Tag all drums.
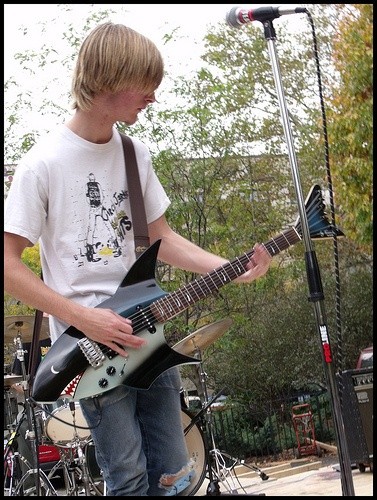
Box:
[44,401,92,449]
[83,406,210,495]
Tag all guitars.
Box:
[31,185,345,404]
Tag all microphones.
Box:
[226,7,307,29]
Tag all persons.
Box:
[4,22,272,497]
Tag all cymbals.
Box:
[171,317,233,354]
[4,315,51,343]
[4,376,27,386]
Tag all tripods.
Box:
[189,336,269,495]
[4,325,104,496]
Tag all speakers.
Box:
[335,366,375,466]
